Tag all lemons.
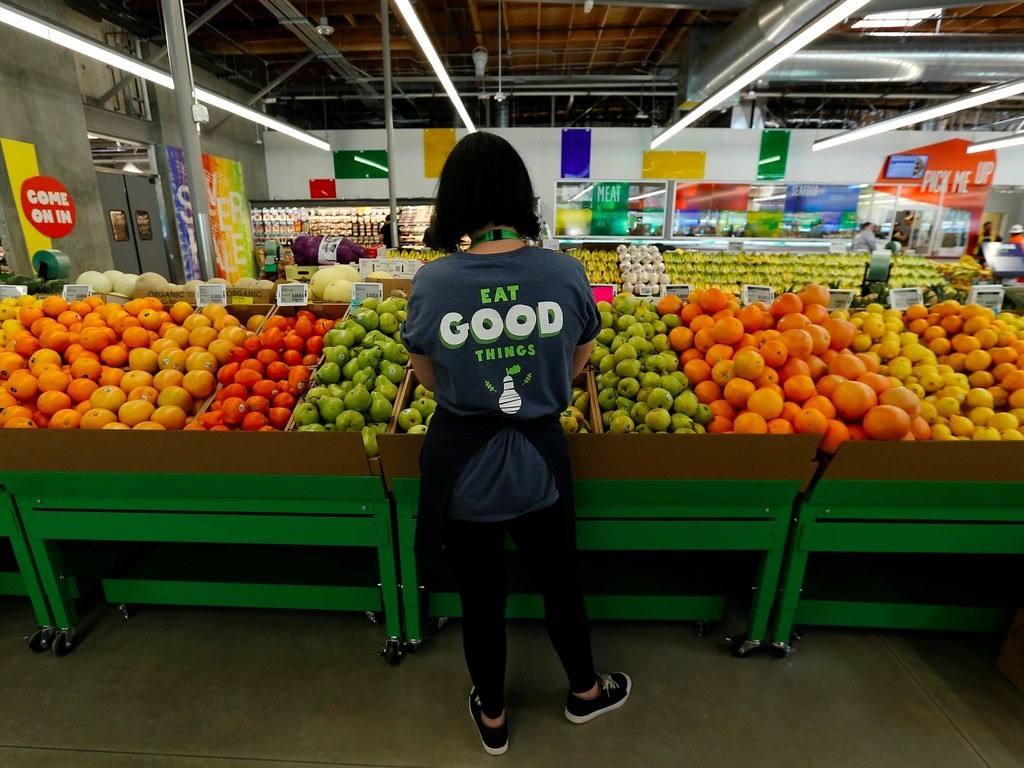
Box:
[829,310,1024,441]
[866,303,884,314]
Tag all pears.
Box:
[559,293,713,434]
[293,297,436,458]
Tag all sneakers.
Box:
[468,683,507,755]
[565,671,632,724]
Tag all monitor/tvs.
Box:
[885,155,929,179]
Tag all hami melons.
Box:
[76,265,392,303]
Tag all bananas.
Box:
[387,249,445,263]
[557,249,956,297]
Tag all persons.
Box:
[851,223,880,254]
[380,213,402,249]
[1003,224,1023,287]
[399,133,631,754]
[973,220,1003,265]
[686,227,697,236]
[808,218,828,238]
[891,212,913,246]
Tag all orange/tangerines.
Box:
[657,285,928,451]
[0,295,339,431]
[903,300,1024,435]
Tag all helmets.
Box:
[1008,223,1024,233]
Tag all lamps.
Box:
[0,0,1024,172]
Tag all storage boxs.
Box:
[163,303,414,433]
[570,359,605,434]
